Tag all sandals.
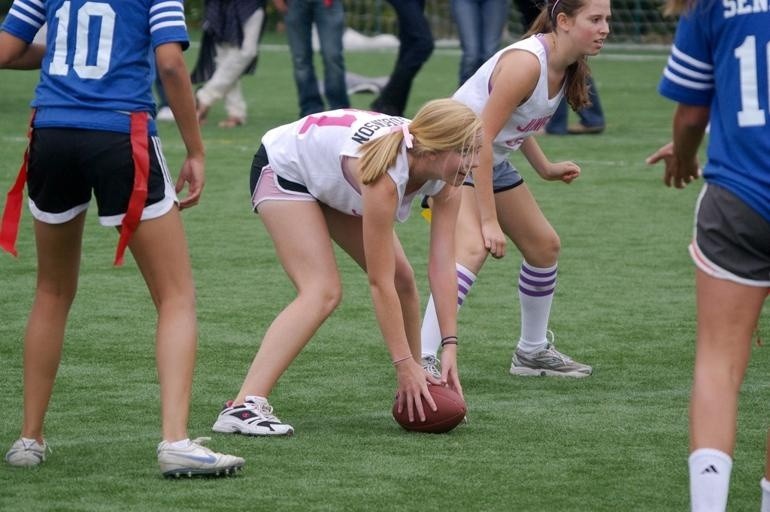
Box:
[217,118,248,129]
[193,87,209,124]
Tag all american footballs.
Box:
[393,384,467,433]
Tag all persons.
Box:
[209,98,485,437]
[642,0,770,511]
[416,0,610,383]
[0,1,244,480]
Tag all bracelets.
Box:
[442,340,459,348]
[441,335,458,343]
[391,354,413,367]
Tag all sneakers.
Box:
[420,354,442,384]
[156,440,244,477]
[212,395,293,437]
[565,122,604,135]
[508,342,592,379]
[5,436,48,467]
